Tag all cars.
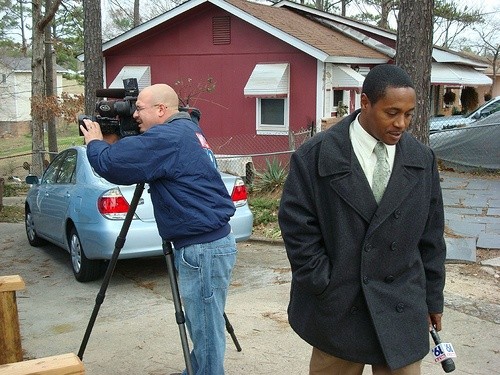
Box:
[428,95,500,133]
[24,147,253,283]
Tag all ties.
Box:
[372,142,390,206]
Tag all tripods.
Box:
[76,183,243,375]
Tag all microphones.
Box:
[429,328,457,373]
[96,89,134,99]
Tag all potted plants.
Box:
[441,91,456,116]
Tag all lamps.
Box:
[353,65,360,73]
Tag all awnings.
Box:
[108,66,151,92]
[244,63,289,97]
[431,62,493,87]
[332,64,365,94]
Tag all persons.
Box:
[278,63,446,375]
[81,83,236,375]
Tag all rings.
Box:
[88,127,92,130]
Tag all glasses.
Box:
[136,104,167,112]
